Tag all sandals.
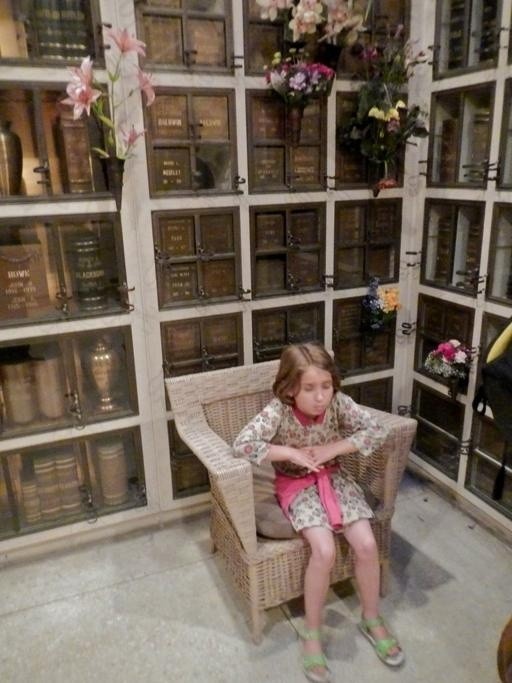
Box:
[355,613,407,667]
[295,616,334,683]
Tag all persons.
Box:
[229,339,405,683]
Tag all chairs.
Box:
[166,352,421,647]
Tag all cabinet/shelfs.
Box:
[0,1,406,534]
[410,2,512,545]
